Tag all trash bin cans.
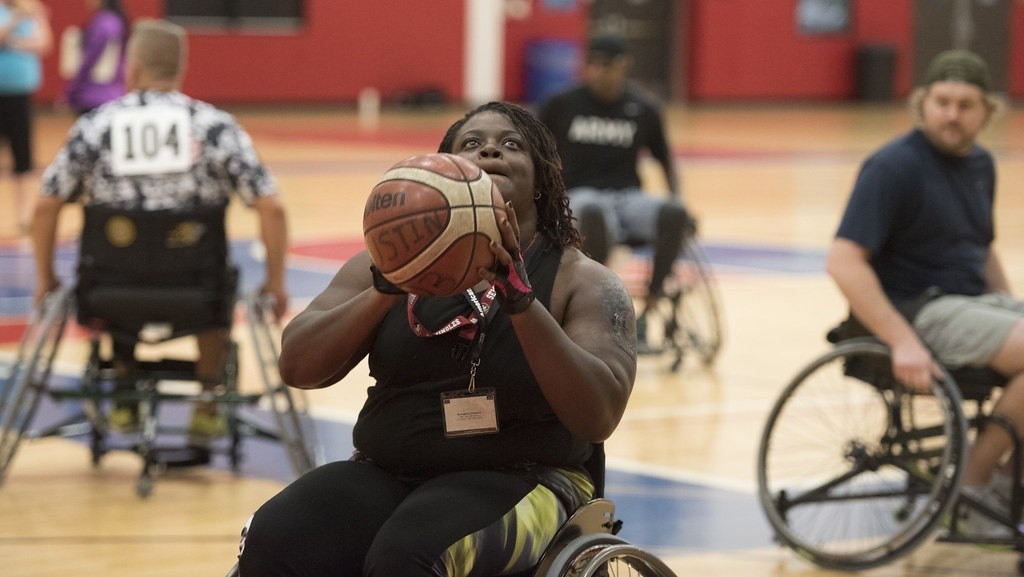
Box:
[854,42,896,103]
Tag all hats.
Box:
[924,49,997,88]
[585,36,624,57]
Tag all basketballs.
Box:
[360,153,510,297]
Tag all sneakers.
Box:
[104,407,138,430]
[188,415,226,433]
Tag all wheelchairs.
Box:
[756,310,1024,572]
[0,189,317,481]
[530,441,675,577]
[635,218,724,376]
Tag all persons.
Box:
[58,0,129,120]
[31,18,291,450]
[0,1,54,238]
[534,34,696,355]
[232,102,637,577]
[826,47,1024,528]
